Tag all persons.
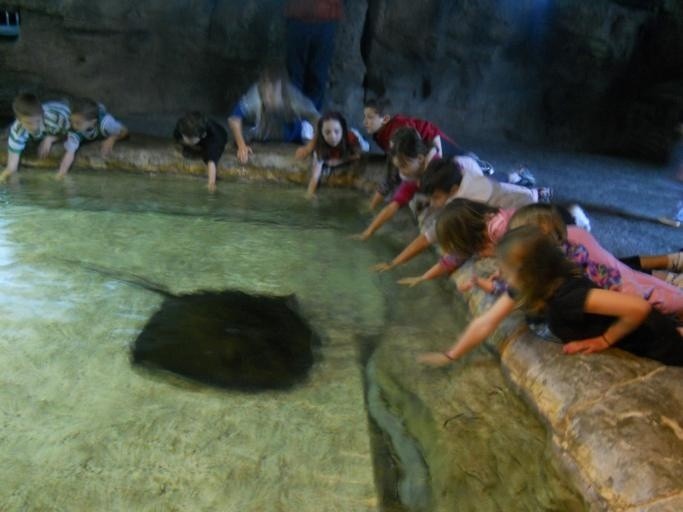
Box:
[457,205,682,334]
[305,108,369,203]
[231,73,323,168]
[345,137,556,243]
[359,125,555,213]
[369,159,593,273]
[395,198,683,319]
[616,249,682,288]
[0,92,72,186]
[172,110,229,194]
[362,96,495,180]
[415,223,683,374]
[50,96,128,175]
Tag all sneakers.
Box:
[476,156,494,174]
[569,202,591,231]
[539,186,554,205]
[515,166,535,187]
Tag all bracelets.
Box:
[386,263,395,267]
[442,351,454,361]
[602,333,611,348]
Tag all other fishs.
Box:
[66,258,318,395]
